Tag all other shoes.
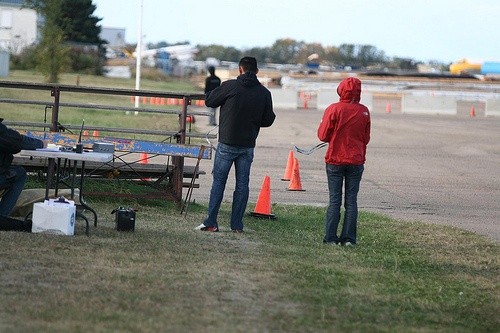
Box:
[344,242,352,246]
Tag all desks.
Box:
[11,151,112,235]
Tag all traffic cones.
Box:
[143,96,184,106]
[139,152,150,180]
[196,99,205,107]
[386,103,392,112]
[280,149,306,191]
[82,128,99,138]
[470,106,476,117]
[131,96,135,105]
[249,176,276,220]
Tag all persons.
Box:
[194,57,276,233]
[0,118,36,230]
[318,77,371,243]
[206,66,221,126]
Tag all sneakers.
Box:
[194,223,219,232]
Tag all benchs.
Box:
[5,154,209,190]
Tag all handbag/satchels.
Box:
[111,207,136,232]
[32,196,76,237]
